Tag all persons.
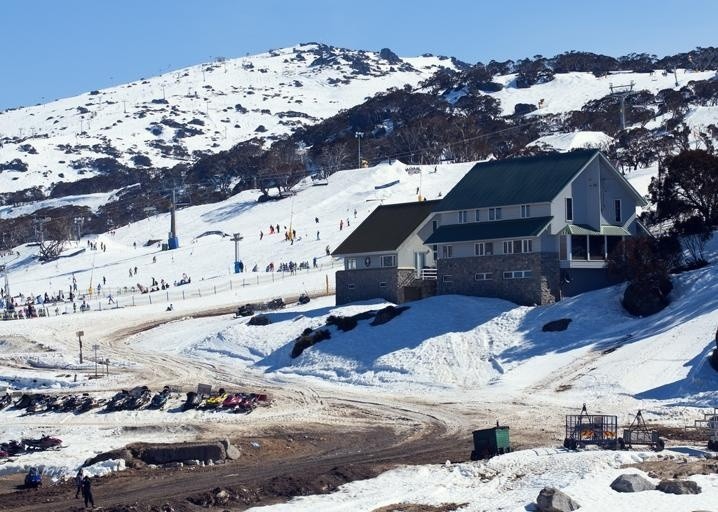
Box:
[133,242,136,249]
[17,251,20,256]
[69,273,78,293]
[82,475,95,508]
[326,246,330,256]
[315,217,319,223]
[340,220,343,230]
[86,228,116,253]
[0,288,114,321]
[439,192,442,196]
[157,241,160,248]
[354,209,357,217]
[167,304,174,311]
[129,267,192,294]
[75,467,87,500]
[317,231,320,239]
[252,257,317,275]
[416,187,427,201]
[259,224,296,245]
[435,167,437,172]
[153,255,157,263]
[102,276,106,284]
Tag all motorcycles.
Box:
[181,387,262,415]
[1,394,97,417]
[24,467,43,490]
[102,386,173,413]
[0,434,69,462]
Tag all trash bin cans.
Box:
[162,244,167,251]
[168,237,179,249]
[471,426,510,457]
